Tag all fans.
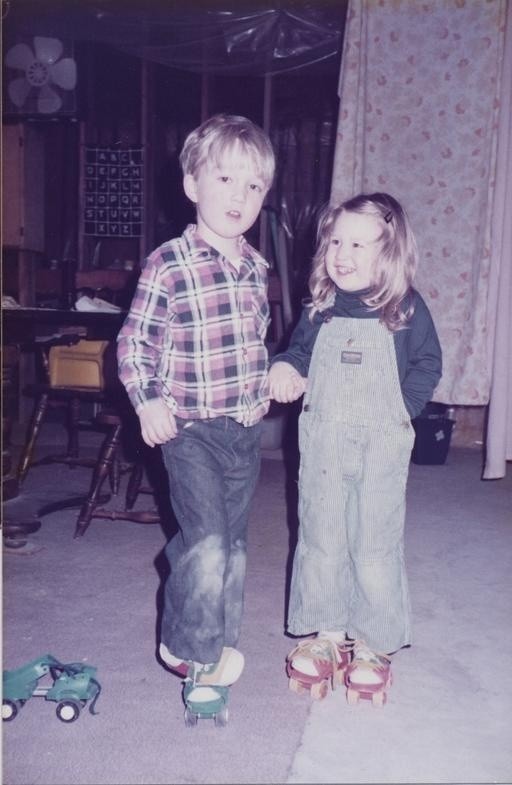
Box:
[4,36,81,115]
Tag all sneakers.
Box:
[180,675,232,728]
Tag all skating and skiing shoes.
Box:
[2,654,103,724]
[286,635,352,702]
[342,651,396,709]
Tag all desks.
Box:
[3,305,128,504]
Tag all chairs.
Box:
[16,268,179,535]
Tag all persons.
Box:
[267,192,443,686]
[117,111,305,690]
